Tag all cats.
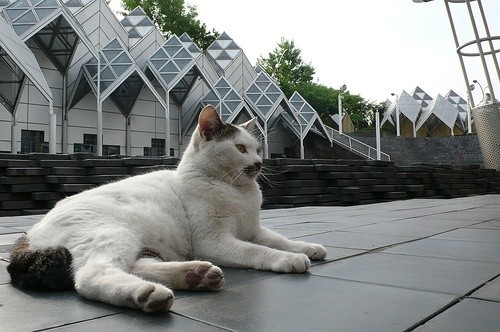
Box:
[7,105,328,314]
